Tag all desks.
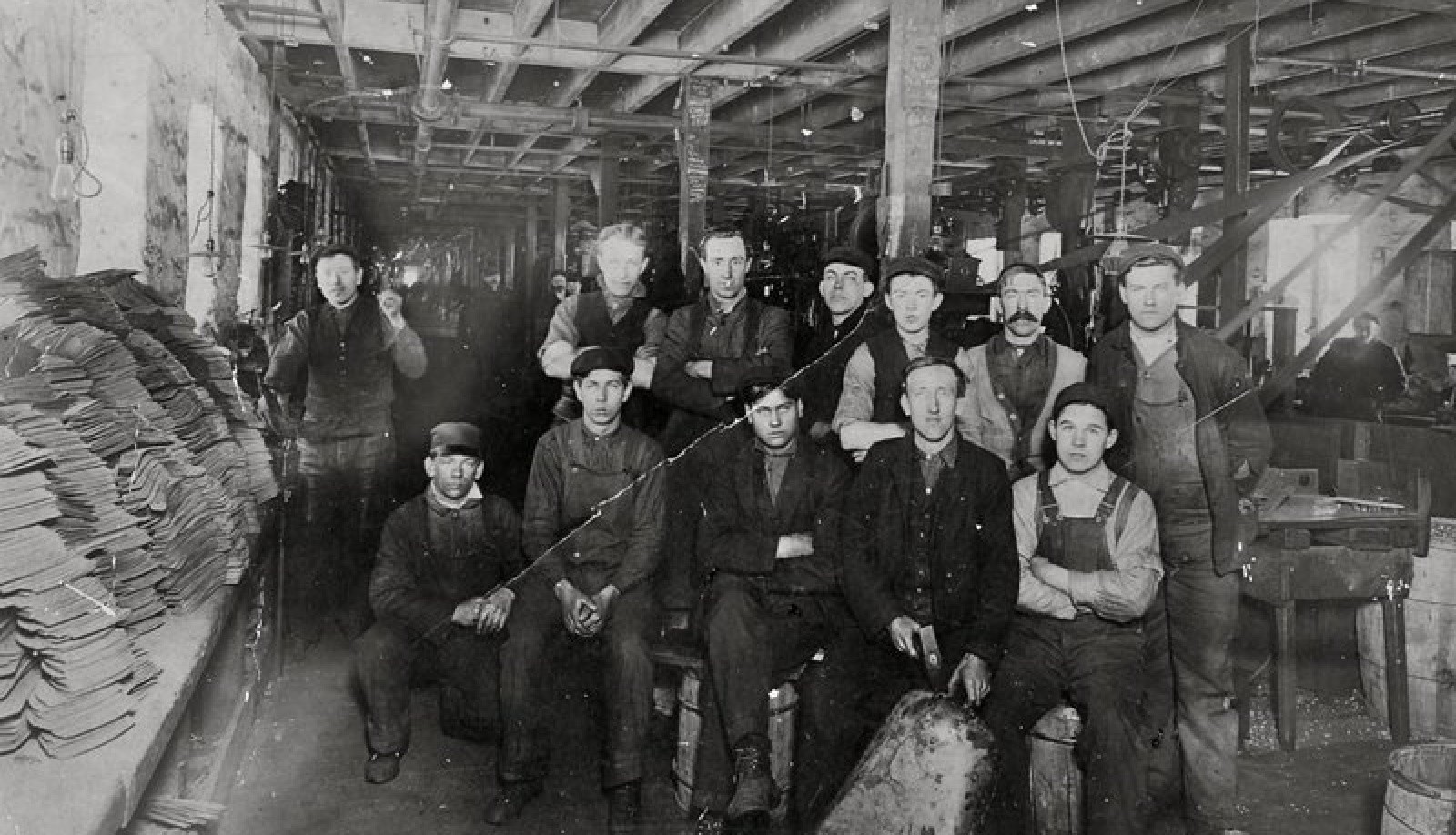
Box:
[1242,494,1426,752]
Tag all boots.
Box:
[727,739,772,826]
[696,812,730,835]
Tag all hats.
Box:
[571,347,635,378]
[887,256,947,287]
[734,364,808,408]
[428,422,483,458]
[1053,381,1120,431]
[821,247,875,276]
[1117,243,1185,275]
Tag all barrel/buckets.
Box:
[1026,704,1085,835]
[671,671,800,828]
[1353,517,1456,744]
[1382,741,1456,835]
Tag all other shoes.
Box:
[609,779,642,835]
[343,613,365,641]
[363,752,407,784]
[303,615,325,644]
[484,777,544,824]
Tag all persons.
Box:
[263,246,426,657]
[351,223,1273,834]
[1310,314,1408,421]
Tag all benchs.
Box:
[648,645,1085,835]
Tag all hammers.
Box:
[916,625,943,691]
